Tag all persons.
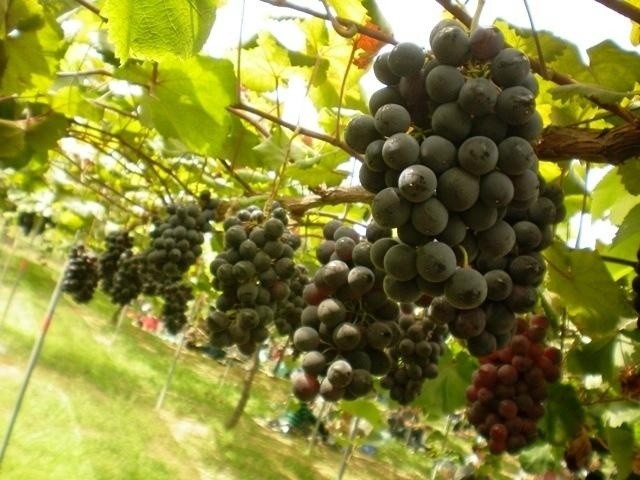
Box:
[395,405,432,454]
[455,443,489,479]
[287,402,343,455]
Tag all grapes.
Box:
[58,20,563,455]
